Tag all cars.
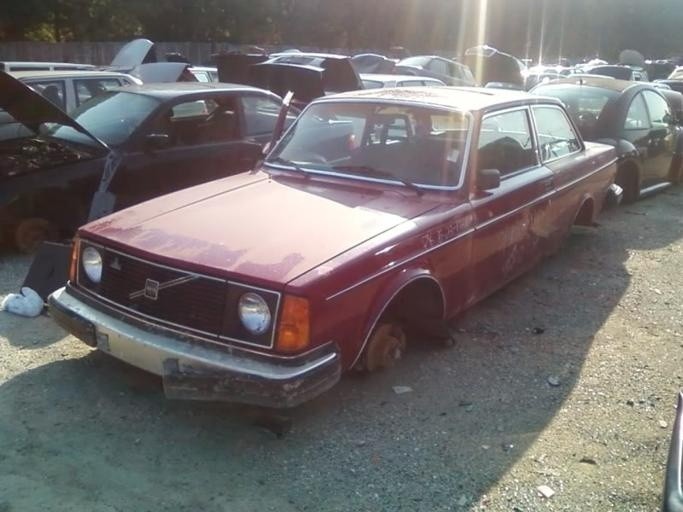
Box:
[528,77,683,203]
[47,86,621,410]
[0,69,363,255]
[1,36,683,122]
